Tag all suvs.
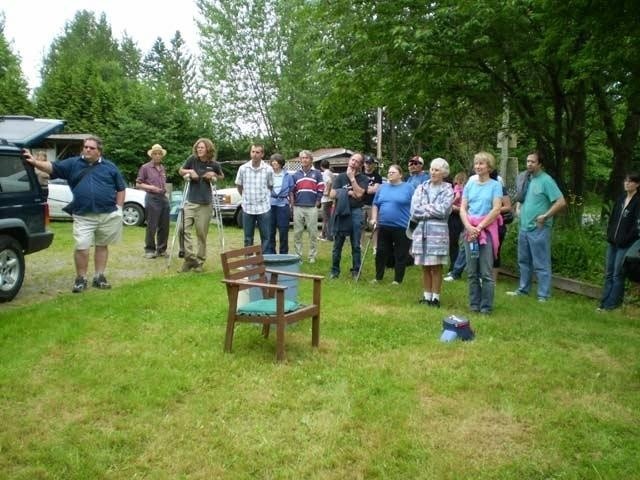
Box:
[0,118,65,304]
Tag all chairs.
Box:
[220,244,325,361]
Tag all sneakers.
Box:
[309,257,315,264]
[443,271,457,281]
[72,273,112,292]
[144,252,173,259]
[177,259,202,273]
[329,271,400,285]
[419,297,440,308]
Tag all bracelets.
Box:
[543,215,547,219]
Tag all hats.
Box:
[147,143,167,158]
[408,155,425,165]
[364,156,373,163]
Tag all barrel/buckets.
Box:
[247,255,301,303]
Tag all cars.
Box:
[213,170,347,230]
[0,168,147,228]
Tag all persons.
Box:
[175,137,224,274]
[329,152,455,309]
[22,137,126,293]
[34,151,50,203]
[444,152,512,313]
[593,171,640,316]
[505,151,568,306]
[135,144,170,259]
[234,144,335,264]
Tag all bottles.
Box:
[469,239,479,258]
